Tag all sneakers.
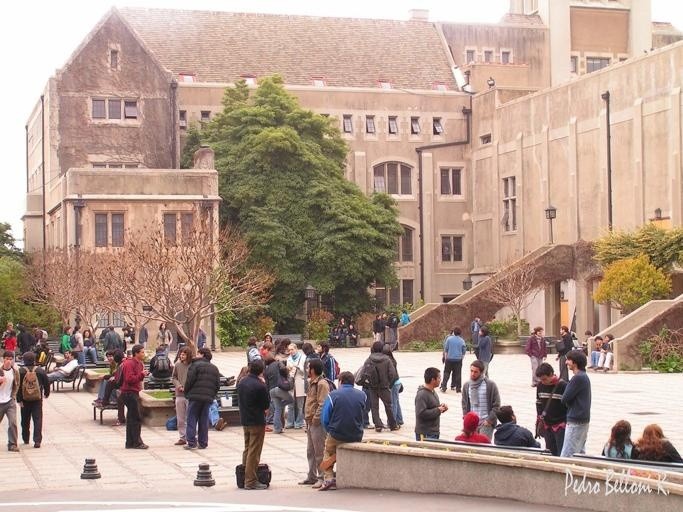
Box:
[265,420,308,434]
[93,398,110,408]
[244,483,268,490]
[319,453,336,471]
[298,479,336,491]
[125,444,148,450]
[215,417,227,431]
[174,439,207,451]
[7,446,20,452]
[23,436,29,445]
[34,442,40,449]
[111,420,125,427]
[439,386,462,393]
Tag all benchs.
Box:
[17,342,238,426]
[517,335,558,354]
[468,336,499,354]
[272,334,304,349]
[422,436,683,474]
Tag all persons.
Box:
[0,311,683,492]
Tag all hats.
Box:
[264,332,272,339]
[464,411,480,433]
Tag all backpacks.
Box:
[555,338,566,352]
[323,354,340,380]
[68,333,78,349]
[391,316,398,327]
[21,365,41,402]
[36,329,48,342]
[153,355,169,374]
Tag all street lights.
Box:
[544,205,556,242]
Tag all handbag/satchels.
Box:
[353,366,364,387]
[277,376,295,391]
[235,464,271,489]
[536,417,547,438]
[474,349,494,363]
[58,343,62,354]
[165,415,178,431]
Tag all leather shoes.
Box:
[364,423,404,432]
[531,383,538,387]
[587,366,611,372]
